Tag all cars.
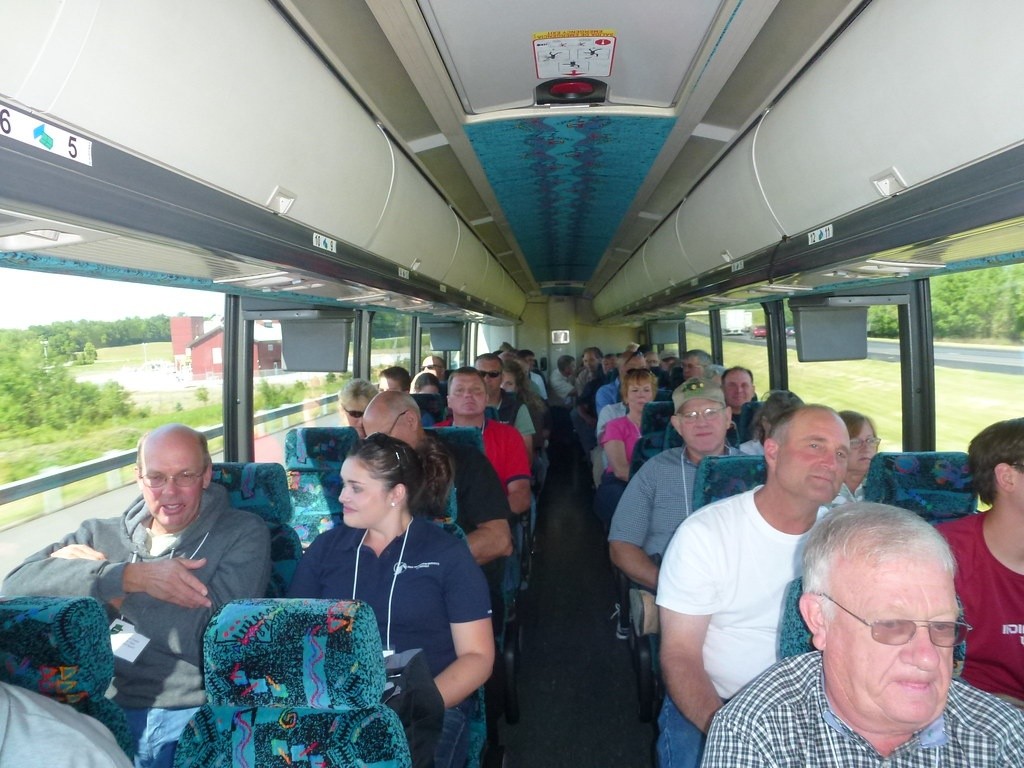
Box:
[786,327,795,337]
[753,325,767,339]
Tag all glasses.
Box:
[478,370,502,379]
[850,436,881,450]
[343,407,364,418]
[819,592,973,648]
[364,432,404,484]
[625,350,643,365]
[420,365,443,372]
[678,406,726,423]
[627,368,654,376]
[138,466,207,488]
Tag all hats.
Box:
[671,374,725,416]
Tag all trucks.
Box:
[741,312,752,332]
[720,309,745,336]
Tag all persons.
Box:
[0,680,134,768]
[361,390,514,635]
[570,338,760,532]
[409,341,548,448]
[283,430,498,768]
[928,415,1023,714]
[697,500,1024,768]
[0,425,275,768]
[338,378,379,440]
[829,409,883,507]
[378,366,411,394]
[548,355,578,451]
[606,376,754,595]
[430,365,532,515]
[739,389,804,456]
[653,402,851,768]
[475,352,536,461]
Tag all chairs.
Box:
[620,390,982,722]
[410,392,447,423]
[283,426,359,550]
[169,597,413,768]
[211,463,304,598]
[425,424,527,724]
[429,482,506,768]
[0,594,136,768]
